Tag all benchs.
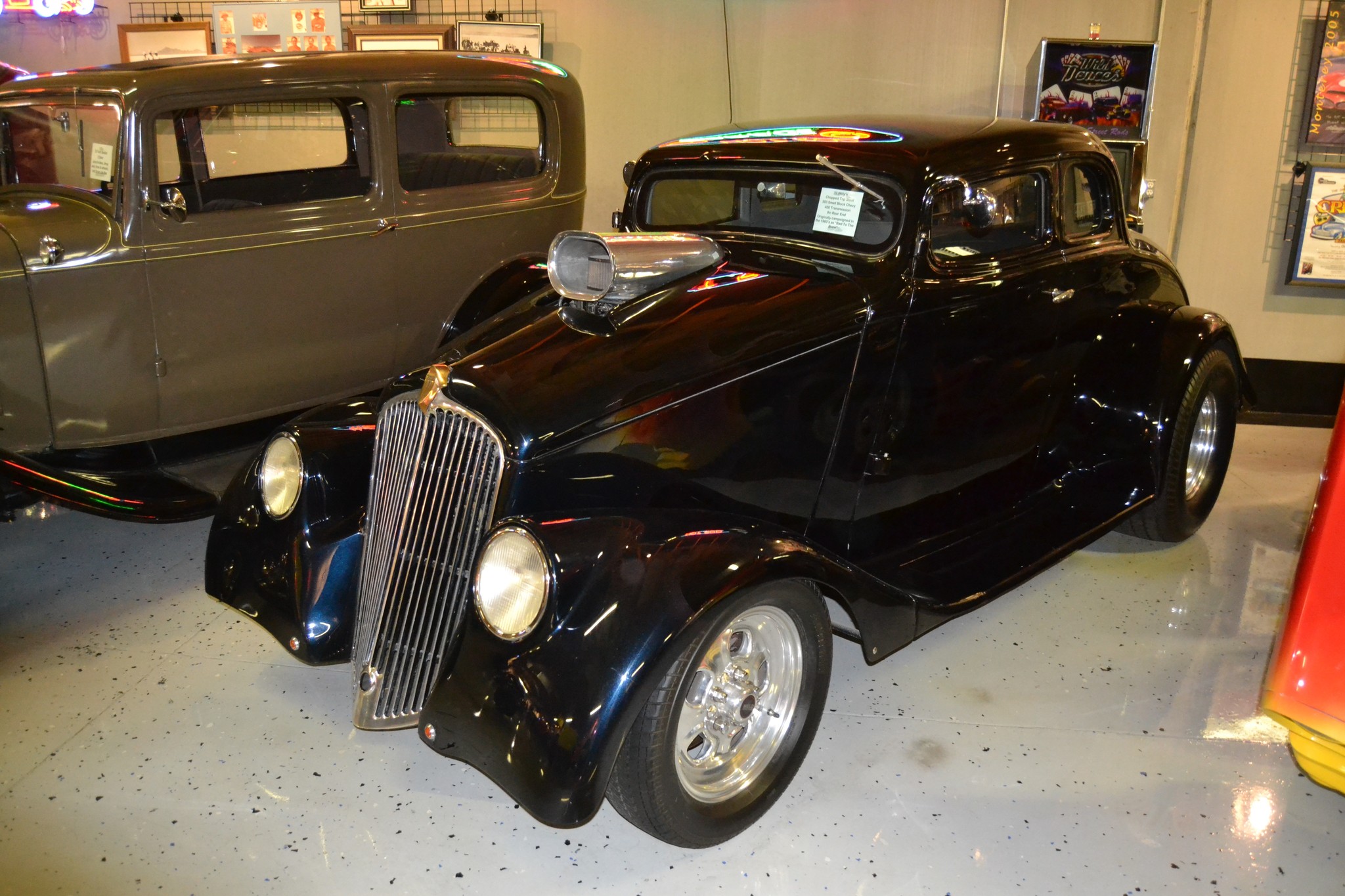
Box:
[399,152,531,190]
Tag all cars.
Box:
[0,45,588,512]
[202,113,1264,856]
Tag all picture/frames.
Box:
[1284,161,1345,287]
[1033,37,1159,141]
